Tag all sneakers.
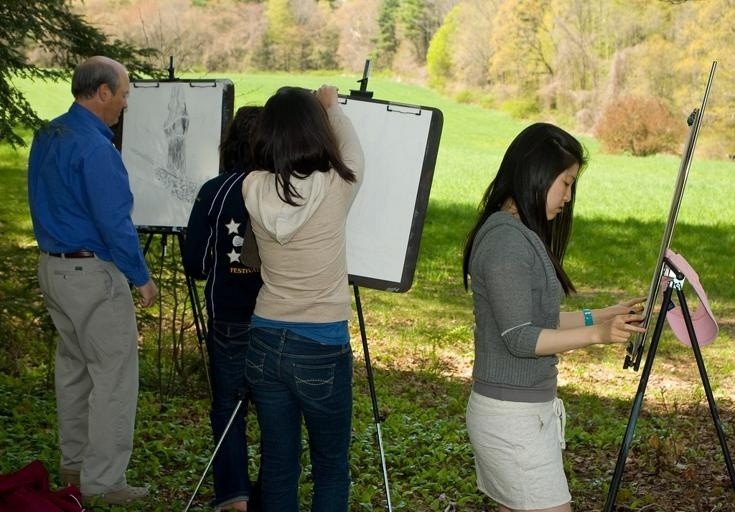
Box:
[58,465,149,507]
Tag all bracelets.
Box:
[582,308,594,327]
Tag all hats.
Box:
[660,248,721,350]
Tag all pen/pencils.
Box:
[625,311,635,325]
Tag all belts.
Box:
[41,249,95,259]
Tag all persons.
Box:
[180,105,272,511]
[240,81,369,511]
[459,122,649,511]
[26,54,160,505]
